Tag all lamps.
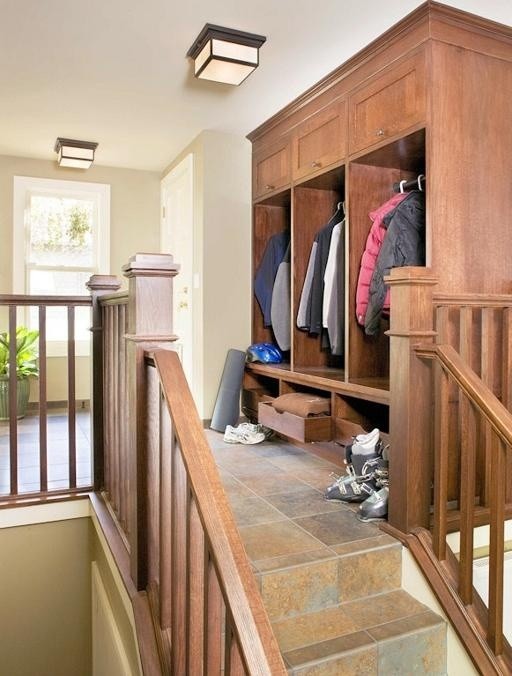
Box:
[185,21,267,88]
[51,136,98,171]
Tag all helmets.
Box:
[246,342,283,364]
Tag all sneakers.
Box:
[222,424,265,445]
[238,421,262,432]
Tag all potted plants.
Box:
[0,325,39,421]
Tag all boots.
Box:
[357,443,390,520]
[326,441,382,502]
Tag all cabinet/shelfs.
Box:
[240,0,512,503]
